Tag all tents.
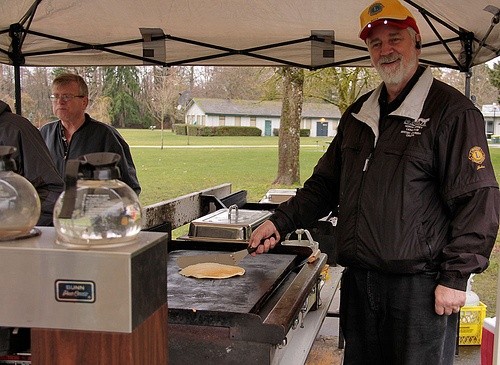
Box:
[0,1,500,116]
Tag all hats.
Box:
[357,0,416,38]
[359,16,420,40]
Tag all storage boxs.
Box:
[480,317,497,365]
[459,302,488,346]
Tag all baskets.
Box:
[458,300,487,346]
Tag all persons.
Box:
[0,99,65,354]
[39,74,141,196]
[248,0,500,365]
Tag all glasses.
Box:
[48,94,85,101]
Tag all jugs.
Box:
[52,153,143,248]
[0,145,41,240]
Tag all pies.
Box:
[179,262,245,279]
